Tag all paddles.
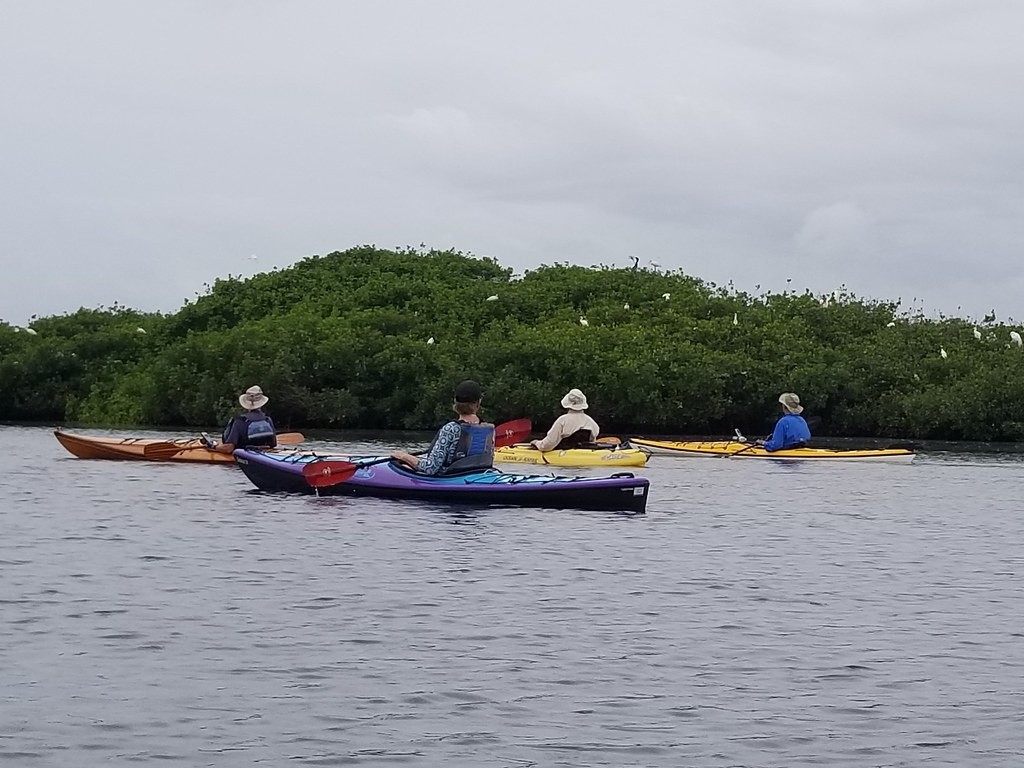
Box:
[597,435,621,446]
[142,433,302,462]
[304,418,533,486]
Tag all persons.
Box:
[207,385,277,453]
[391,379,495,475]
[755,392,811,452]
[530,389,599,451]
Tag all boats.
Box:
[492,444,654,467]
[627,435,918,465]
[53,426,349,464]
[233,447,651,513]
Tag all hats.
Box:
[455,380,487,403]
[239,385,268,409]
[778,392,804,414]
[561,389,588,410]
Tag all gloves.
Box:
[755,438,766,446]
[207,440,219,450]
